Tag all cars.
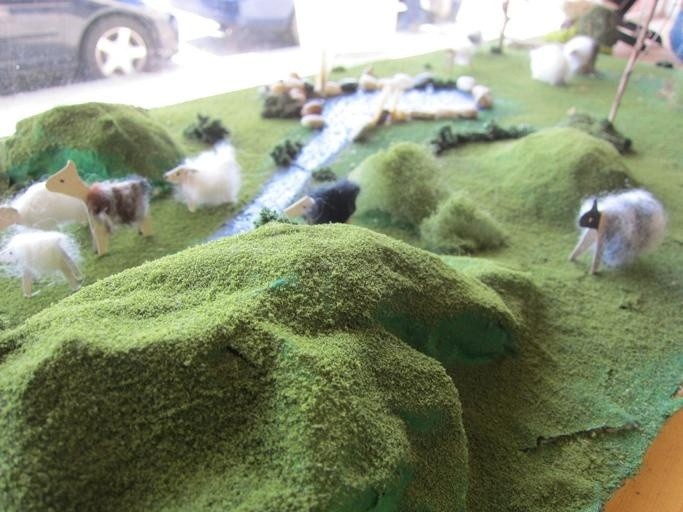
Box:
[1,0,179,98]
